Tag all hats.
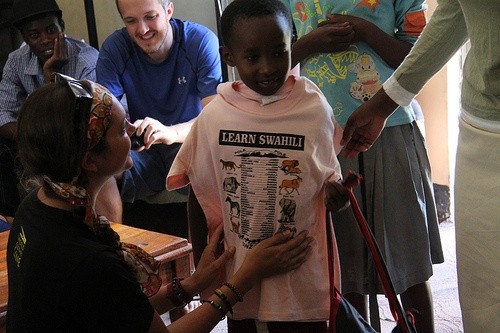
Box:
[15,0,62,26]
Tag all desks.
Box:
[0,221,197,333]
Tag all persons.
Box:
[280,0,444,333]
[340,0,500,333]
[96,0,223,228]
[4,73,314,333]
[165,0,359,333]
[0,0,130,143]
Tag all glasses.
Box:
[49,71,94,163]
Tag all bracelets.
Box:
[223,281,243,302]
[214,288,234,319]
[171,125,179,144]
[206,296,226,320]
[170,277,193,309]
